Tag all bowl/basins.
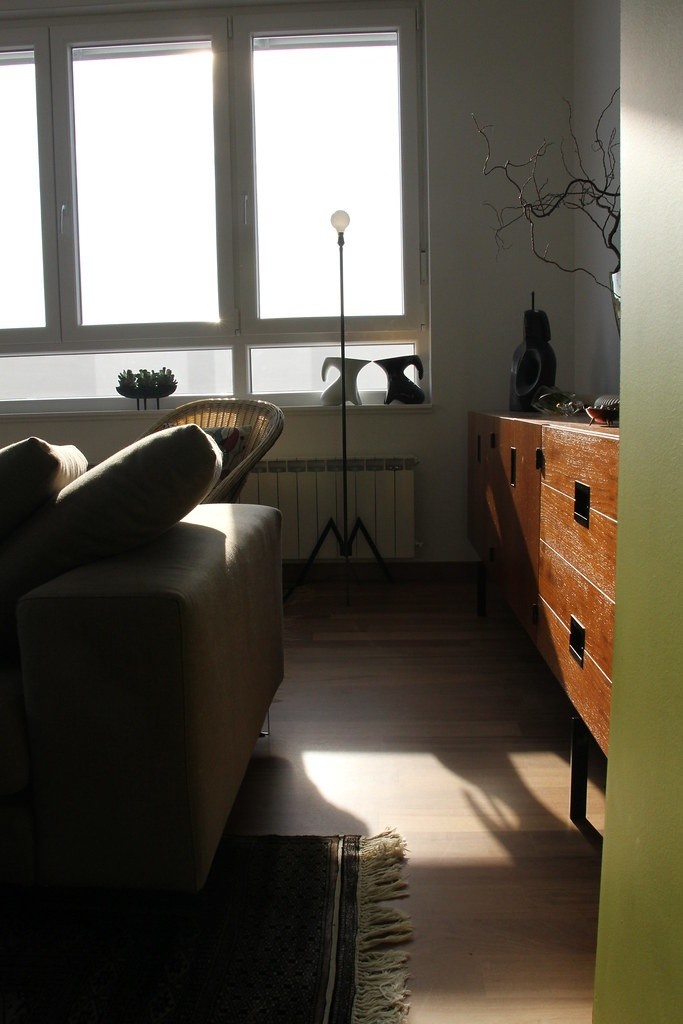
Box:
[586,408,619,423]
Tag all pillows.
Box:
[11,423,223,594]
[0,434,89,545]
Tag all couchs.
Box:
[1,424,286,904]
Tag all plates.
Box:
[594,395,619,406]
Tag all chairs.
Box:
[138,397,286,503]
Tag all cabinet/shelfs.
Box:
[466,409,594,647]
[541,417,620,765]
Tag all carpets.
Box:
[0,832,411,1022]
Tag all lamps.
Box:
[307,210,388,568]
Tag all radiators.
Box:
[238,461,416,571]
